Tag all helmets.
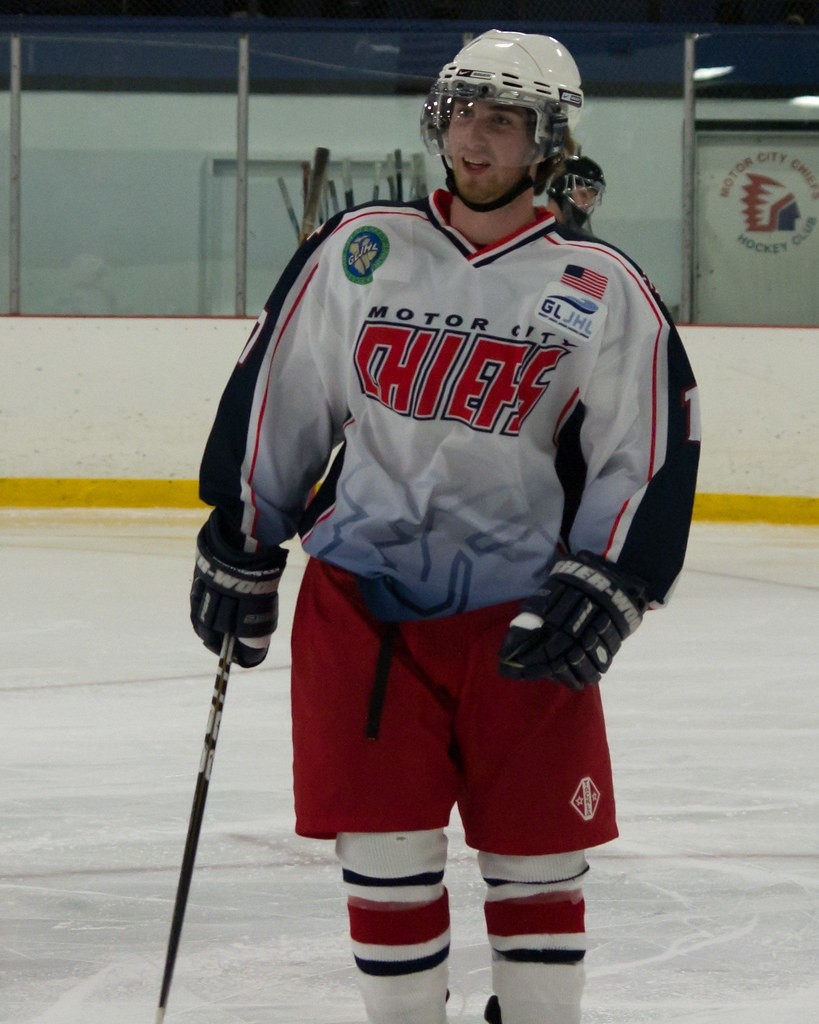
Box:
[547,155,606,201]
[431,29,584,144]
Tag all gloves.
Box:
[188,521,290,669]
[494,549,646,691]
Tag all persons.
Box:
[192,29,700,1021]
[544,156,605,229]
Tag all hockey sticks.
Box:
[149,628,240,1023]
